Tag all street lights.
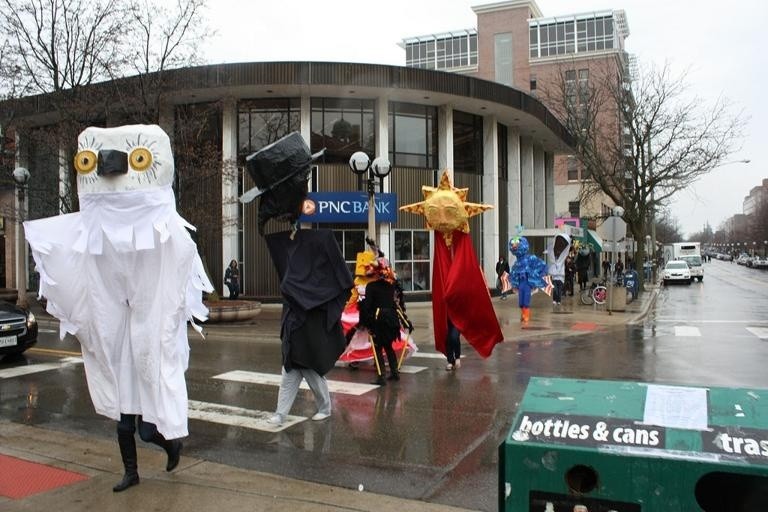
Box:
[646,235,652,282]
[743,242,748,253]
[752,242,757,258]
[763,240,768,255]
[713,241,741,257]
[346,147,395,248]
[11,167,34,310]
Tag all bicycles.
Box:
[580,277,636,308]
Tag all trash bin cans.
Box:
[606,287,626,312]
[624,271,638,300]
[498,377,768,512]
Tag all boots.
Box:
[113,429,140,491]
[143,426,182,471]
[521,307,529,322]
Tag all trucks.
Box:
[663,241,704,281]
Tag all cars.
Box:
[706,248,768,270]
[0,295,40,363]
[662,259,692,285]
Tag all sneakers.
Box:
[312,413,327,421]
[447,358,460,371]
[386,375,400,381]
[371,379,386,385]
[268,416,281,425]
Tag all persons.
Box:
[224,260,240,300]
[336,249,419,371]
[546,232,572,305]
[561,256,577,297]
[357,237,414,385]
[22,124,216,491]
[496,256,509,301]
[237,130,355,429]
[500,224,556,330]
[613,257,623,276]
[601,255,611,278]
[398,171,504,372]
[572,244,591,292]
